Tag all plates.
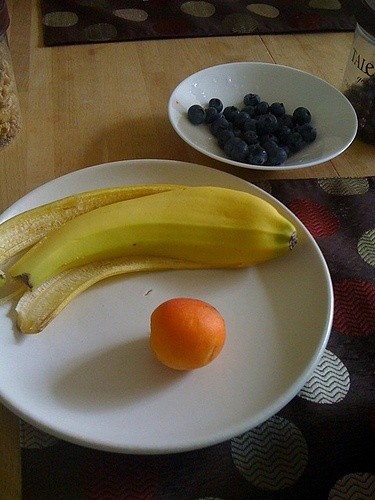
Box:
[0,159,334,455]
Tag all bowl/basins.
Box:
[168,62,358,170]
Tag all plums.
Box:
[149,297,226,370]
